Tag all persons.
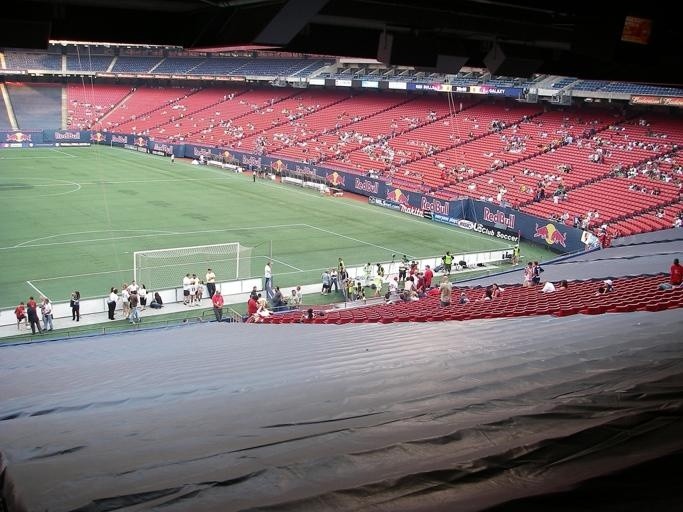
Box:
[459,292,470,304]
[64,86,683,245]
[71,291,80,321]
[481,284,501,301]
[15,296,53,334]
[320,251,455,307]
[301,308,316,323]
[594,280,614,295]
[512,246,521,267]
[524,261,544,287]
[538,279,568,293]
[658,258,683,290]
[212,291,224,322]
[182,267,216,305]
[264,263,271,287]
[108,280,163,324]
[248,285,302,321]
